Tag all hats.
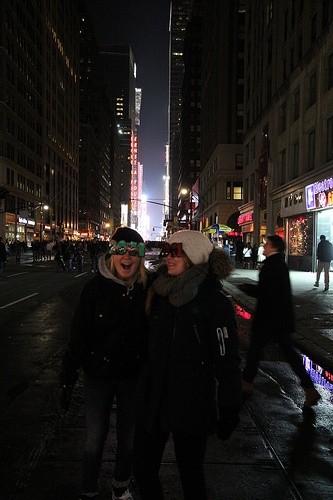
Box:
[165,228,216,266]
[110,225,145,246]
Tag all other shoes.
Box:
[111,478,135,500]
[302,384,321,411]
[239,375,255,397]
[76,489,101,500]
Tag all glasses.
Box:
[159,246,180,259]
[115,245,140,257]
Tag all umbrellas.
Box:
[201,224,232,234]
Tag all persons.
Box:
[314,235,333,291]
[59,227,238,500]
[0,236,65,272]
[224,238,266,270]
[233,235,321,408]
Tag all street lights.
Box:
[39,205,49,243]
[180,187,192,230]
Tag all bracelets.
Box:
[62,384,73,389]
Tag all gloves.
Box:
[214,405,242,441]
[57,368,77,413]
